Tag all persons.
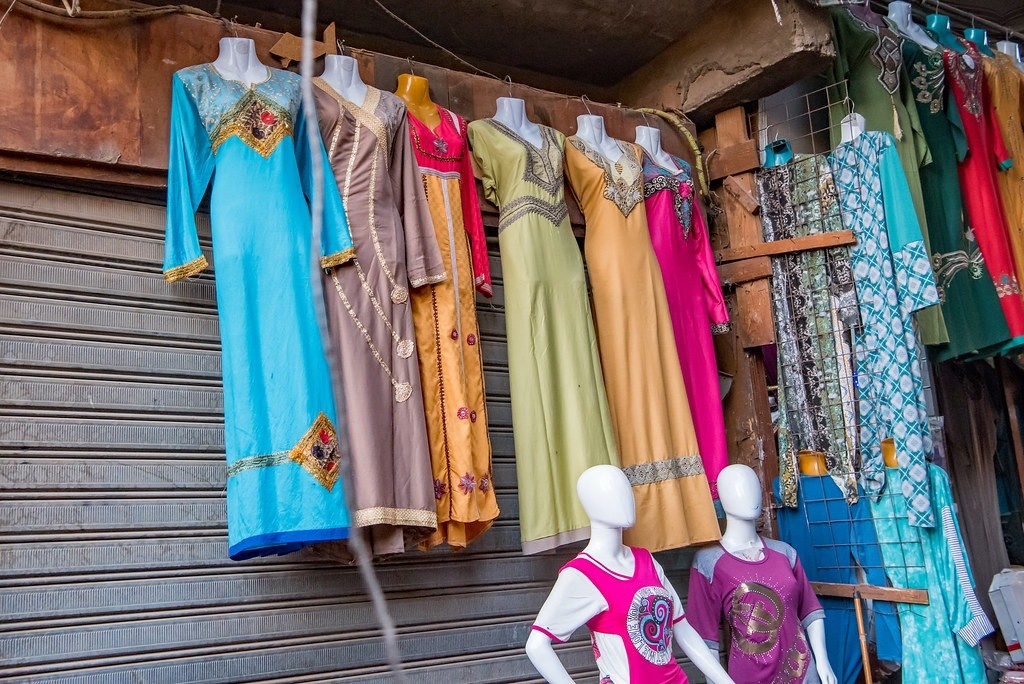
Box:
[526,442,992,684]
[165,0,1024,559]
